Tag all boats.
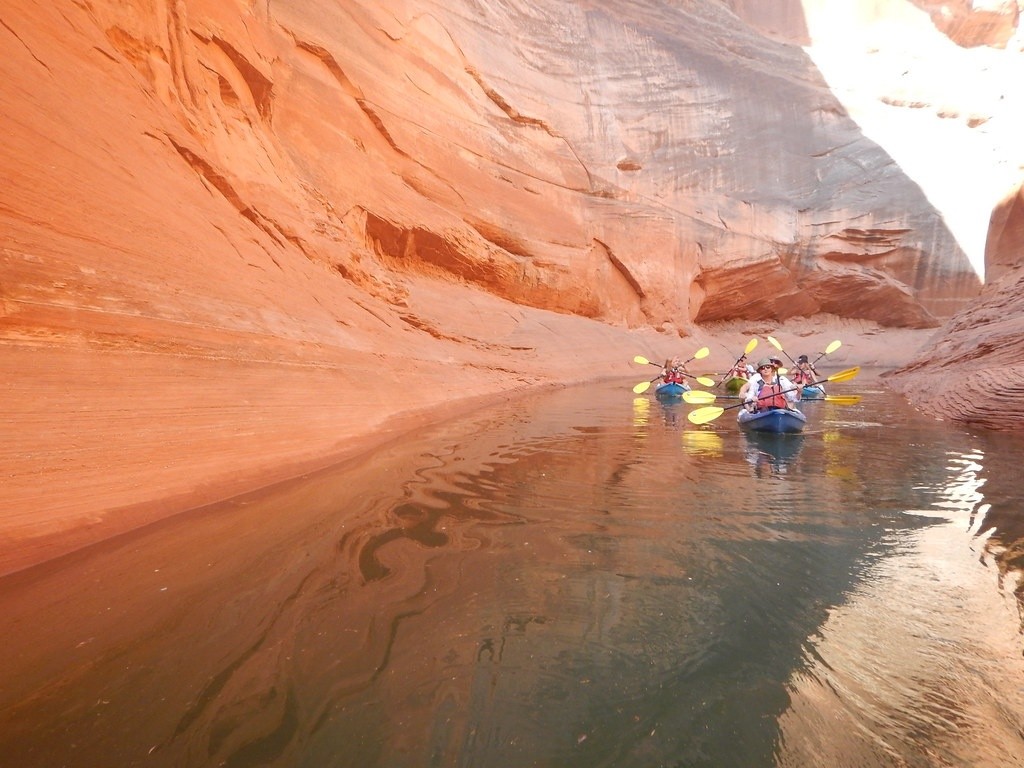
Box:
[725,375,750,393]
[801,383,827,399]
[655,378,693,397]
[737,403,807,433]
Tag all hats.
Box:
[797,358,807,364]
[770,356,783,367]
[756,358,776,373]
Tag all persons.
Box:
[785,355,822,388]
[659,355,690,387]
[728,356,754,380]
[739,357,803,412]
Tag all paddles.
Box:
[681,389,863,406]
[634,354,716,387]
[790,339,842,382]
[632,346,710,395]
[686,365,861,426]
[716,337,759,388]
[766,335,830,397]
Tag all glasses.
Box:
[760,366,770,369]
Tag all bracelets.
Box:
[812,368,816,371]
